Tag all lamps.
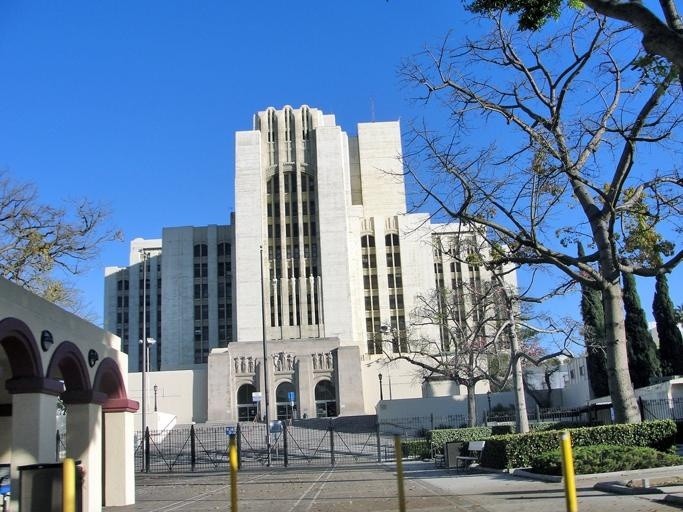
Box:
[40,329,52,351]
[88,349,97,367]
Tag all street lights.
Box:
[137,337,156,372]
[378,373,383,400]
[486,390,493,418]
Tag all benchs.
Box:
[455,440,485,474]
[434,454,445,468]
